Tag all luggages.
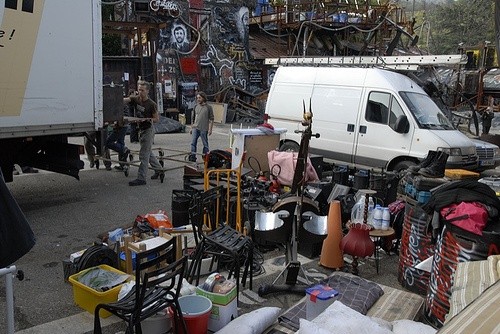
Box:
[381,201,405,255]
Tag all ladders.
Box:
[265,55,468,70]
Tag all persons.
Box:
[85,126,112,170]
[480,106,494,134]
[188,91,215,161]
[106,106,131,171]
[121,80,163,186]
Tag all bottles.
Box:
[356,196,390,231]
[270,176,281,195]
[258,171,268,181]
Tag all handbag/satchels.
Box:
[440,201,490,236]
[267,150,319,185]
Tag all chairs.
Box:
[94,236,188,334]
[186,185,254,307]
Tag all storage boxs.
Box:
[444,169,480,181]
[70,249,88,263]
[305,284,339,322]
[197,285,238,333]
[95,222,133,272]
[69,264,136,319]
[404,172,447,203]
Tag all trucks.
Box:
[0,0,104,181]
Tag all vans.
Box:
[263,62,482,178]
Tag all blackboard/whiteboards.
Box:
[249,70,263,83]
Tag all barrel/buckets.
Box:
[168,293,213,334]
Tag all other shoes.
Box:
[13,171,19,175]
[116,165,128,170]
[89,161,95,168]
[23,168,38,173]
[106,165,112,171]
[128,178,146,186]
[151,172,165,180]
[124,148,131,162]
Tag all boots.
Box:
[418,151,449,177]
[407,150,437,174]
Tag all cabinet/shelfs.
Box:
[232,135,280,176]
[207,101,228,124]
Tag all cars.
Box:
[470,139,500,169]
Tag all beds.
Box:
[263,271,425,334]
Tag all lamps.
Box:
[339,222,375,275]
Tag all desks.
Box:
[346,221,395,274]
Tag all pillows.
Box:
[293,301,438,334]
[212,306,282,334]
